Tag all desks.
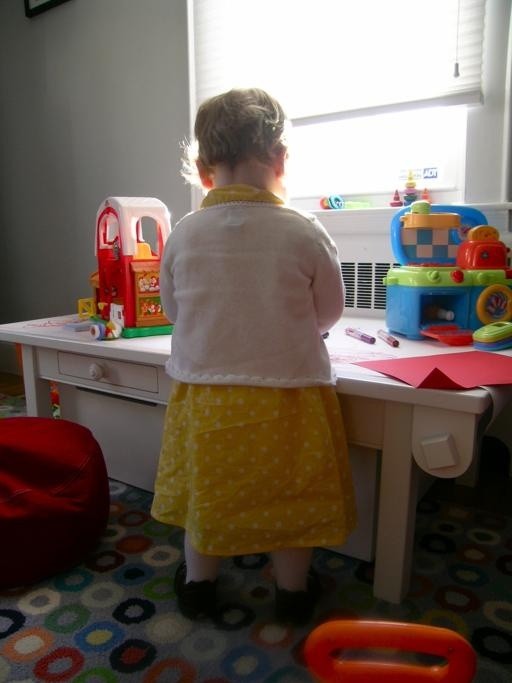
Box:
[2,312,504,604]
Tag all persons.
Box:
[151,89,357,624]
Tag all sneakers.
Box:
[175,557,220,618]
[275,563,321,625]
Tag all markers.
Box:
[346,328,399,347]
